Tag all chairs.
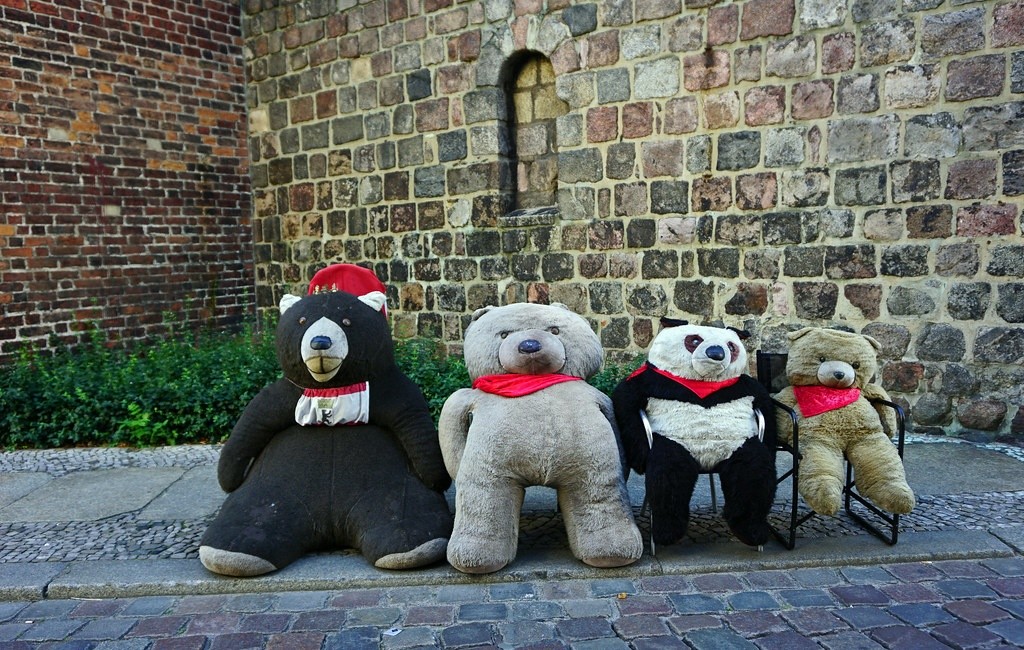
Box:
[639,406,765,556]
[756,349,906,551]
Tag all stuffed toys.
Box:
[613,317,782,552]
[439,302,644,574]
[774,327,915,515]
[198,284,454,577]
